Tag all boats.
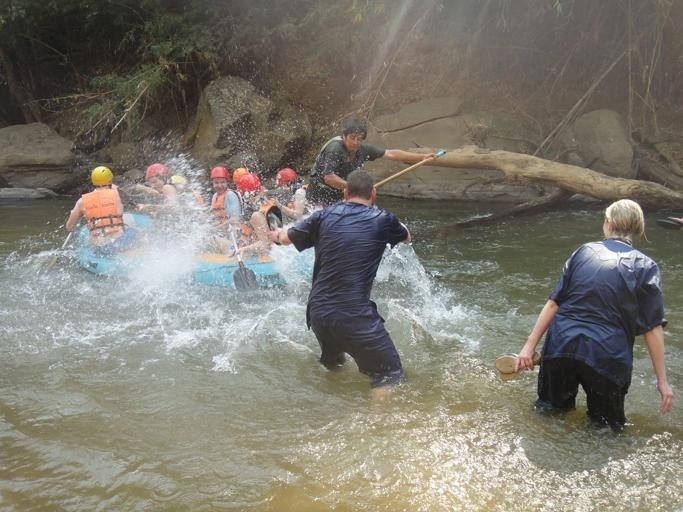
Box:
[69,205,315,296]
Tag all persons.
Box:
[120,163,309,258]
[66,165,143,258]
[305,120,437,213]
[515,198,674,438]
[267,169,411,397]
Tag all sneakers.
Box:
[496,352,541,374]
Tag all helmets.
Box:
[210,167,261,190]
[276,168,298,185]
[146,163,170,179]
[91,166,113,186]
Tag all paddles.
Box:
[47,228,74,269]
[230,229,259,292]
